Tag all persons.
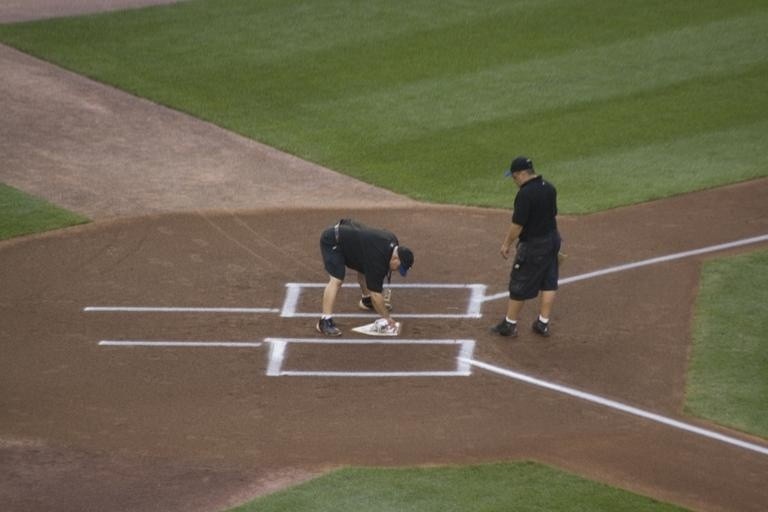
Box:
[315,217,414,336]
[491,156,563,338]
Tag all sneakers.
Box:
[489,318,519,337]
[358,296,392,311]
[316,316,342,337]
[532,316,550,337]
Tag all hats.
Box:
[504,156,533,177]
[397,246,414,277]
